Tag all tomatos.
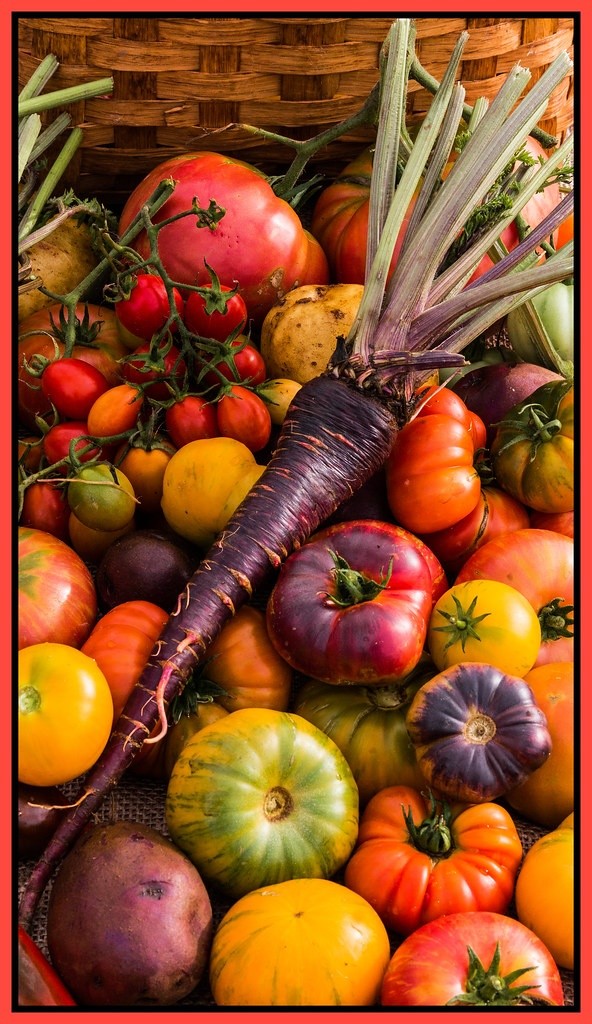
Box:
[16,17,575,1006]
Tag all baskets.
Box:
[18,18,577,196]
